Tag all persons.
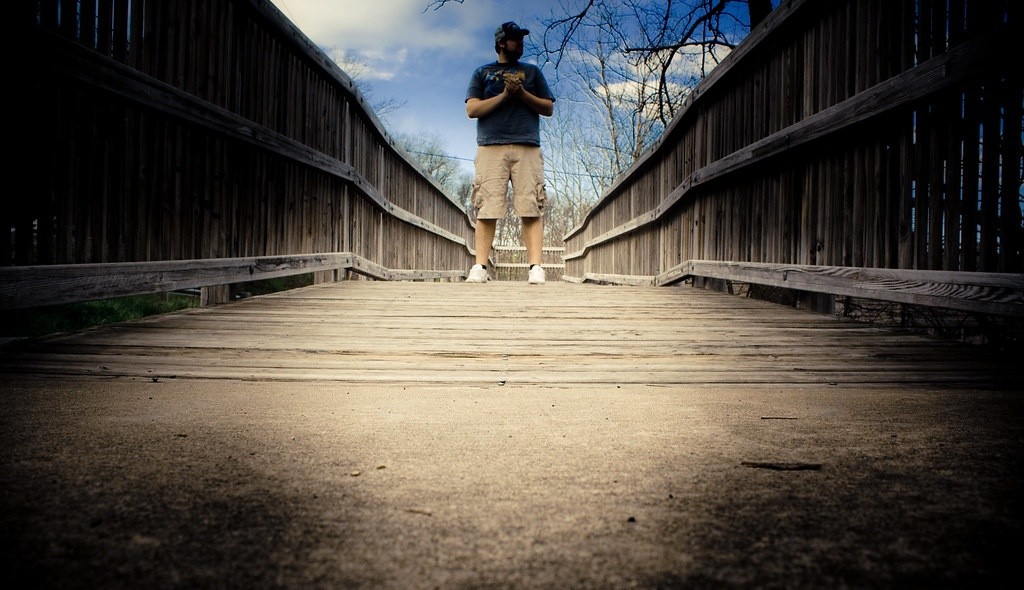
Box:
[465,21,556,284]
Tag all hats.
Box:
[495,21,529,41]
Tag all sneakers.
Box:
[465,264,487,283]
[528,265,545,285]
